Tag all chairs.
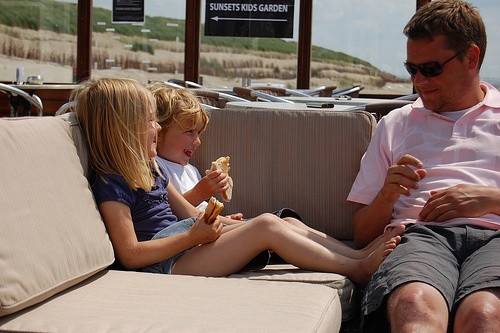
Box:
[163,79,422,123]
[0,83,44,117]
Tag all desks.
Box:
[224,102,367,110]
[9,81,86,116]
[278,97,414,120]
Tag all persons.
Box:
[347,1,499,332]
[69,78,405,289]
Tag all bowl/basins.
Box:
[27,74,43,85]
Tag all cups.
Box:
[16,65,24,85]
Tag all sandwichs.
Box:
[204,196,224,224]
[211,156,234,202]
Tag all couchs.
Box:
[0,105,385,333]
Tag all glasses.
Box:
[404,47,469,77]
